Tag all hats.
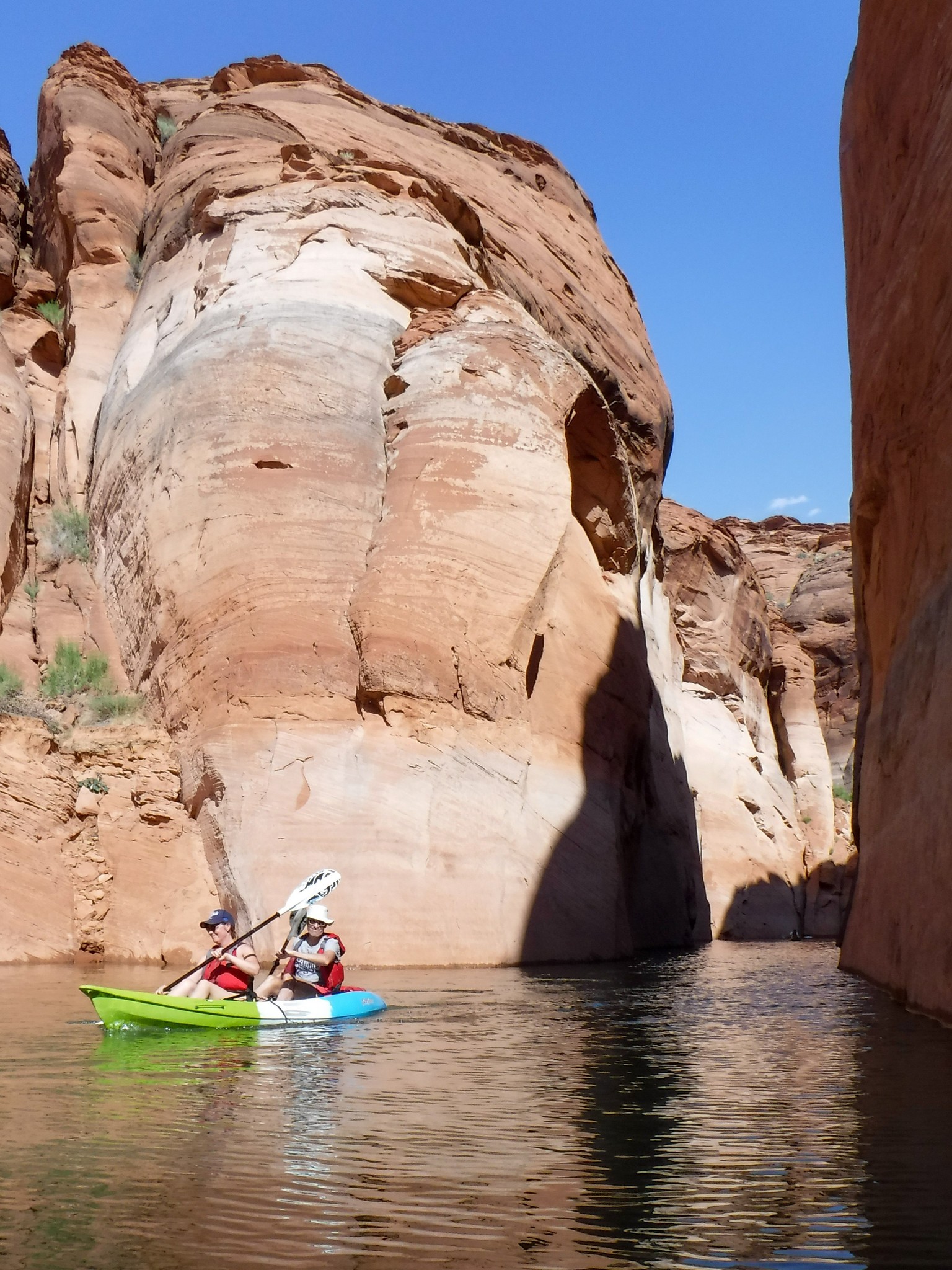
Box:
[200,909,234,928]
[307,905,334,926]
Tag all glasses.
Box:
[308,918,326,927]
[207,923,226,932]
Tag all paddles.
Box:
[151,868,342,992]
[265,909,307,977]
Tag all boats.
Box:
[79,984,388,1036]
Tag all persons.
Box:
[155,905,346,1001]
